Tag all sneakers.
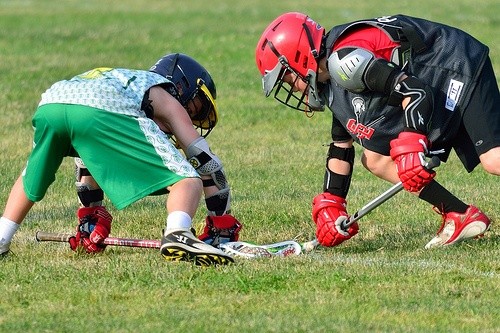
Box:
[425,203,491,247]
[160,229,235,267]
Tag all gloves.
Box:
[67,206,113,255]
[389,131,436,194]
[310,191,360,247]
[198,215,243,245]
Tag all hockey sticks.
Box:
[36,231,276,260]
[265,155,441,258]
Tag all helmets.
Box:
[255,11,325,112]
[150,53,218,129]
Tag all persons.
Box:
[255,10,500,250]
[0,52,242,267]
